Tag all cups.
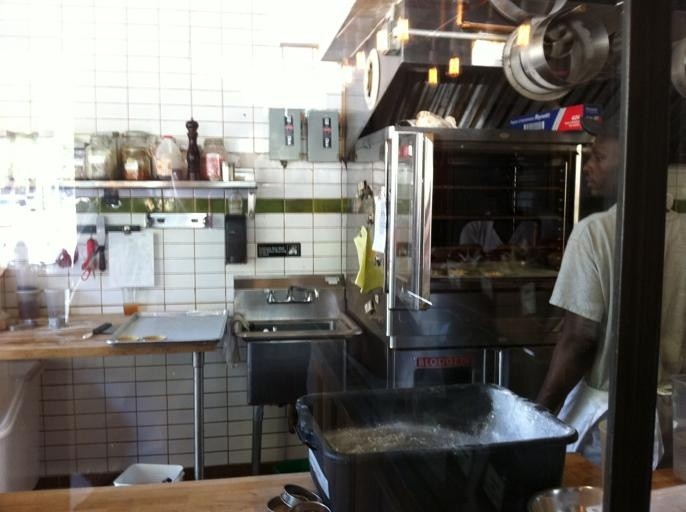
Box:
[121,286,138,316]
[597,419,607,488]
[220,161,234,182]
[42,289,63,328]
[12,258,42,326]
[670,373,686,483]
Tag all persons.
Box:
[535,117,686,469]
[461,196,535,258]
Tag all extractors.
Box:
[364,74,623,132]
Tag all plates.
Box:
[112,333,166,342]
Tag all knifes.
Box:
[82,321,117,341]
[94,214,106,271]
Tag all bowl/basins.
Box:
[526,486,605,512]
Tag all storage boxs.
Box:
[114,464,184,487]
[295,382,577,512]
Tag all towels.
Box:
[218,312,250,368]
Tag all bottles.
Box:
[202,136,226,181]
[184,120,200,181]
[84,130,152,180]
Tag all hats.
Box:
[580,110,621,137]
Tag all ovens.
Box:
[318,310,596,417]
[345,126,605,345]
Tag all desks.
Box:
[0,470,326,512]
[0,315,229,480]
